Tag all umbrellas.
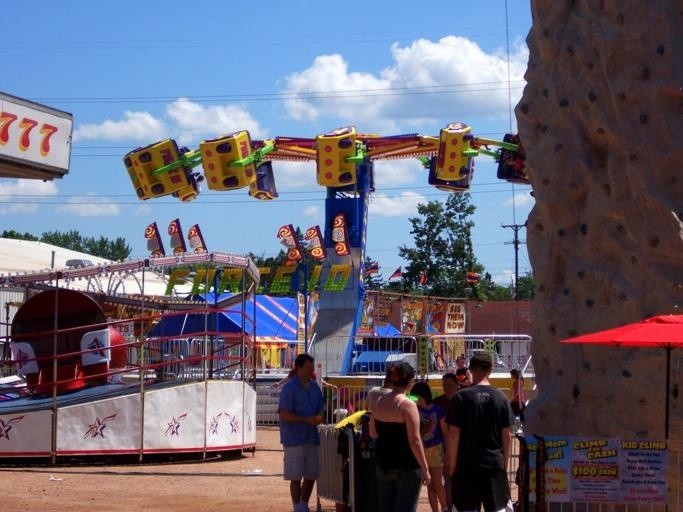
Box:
[559,301,683,439]
